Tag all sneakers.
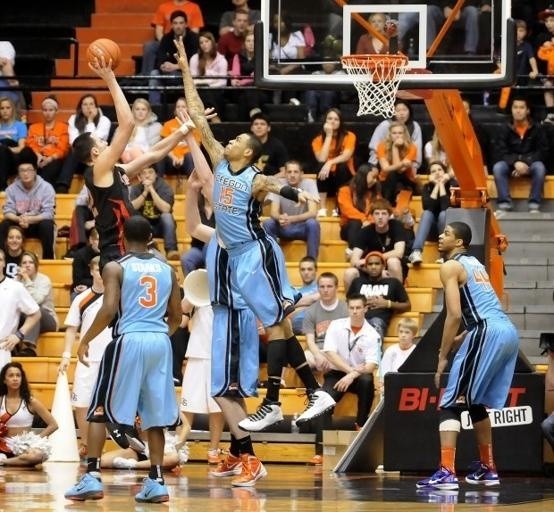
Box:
[295,387,337,427]
[408,250,421,264]
[112,456,136,469]
[125,425,144,452]
[317,208,326,218]
[134,476,168,502]
[231,452,267,487]
[436,258,443,262]
[415,465,459,489]
[20,348,34,356]
[238,397,284,431]
[211,452,242,476]
[206,449,221,463]
[78,442,87,460]
[166,251,179,261]
[105,423,129,448]
[64,473,103,501]
[464,460,500,486]
[306,455,323,465]
[332,208,338,218]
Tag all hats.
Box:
[364,251,385,267]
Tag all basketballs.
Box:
[86,38,120,70]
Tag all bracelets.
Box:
[15,330,24,340]
[179,123,190,136]
[140,193,146,200]
[62,352,71,359]
[279,185,303,203]
[387,300,391,308]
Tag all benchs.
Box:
[1,173,86,288]
[9,288,80,411]
[127,171,188,285]
[261,170,444,287]
[232,360,379,430]
[461,90,554,198]
[34,431,318,462]
[293,288,435,350]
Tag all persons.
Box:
[424,128,459,187]
[154,97,205,178]
[148,0,337,34]
[415,221,519,490]
[0,97,29,191]
[24,95,75,194]
[338,0,553,95]
[72,55,219,451]
[250,113,290,176]
[541,344,554,450]
[64,214,183,503]
[303,272,351,372]
[172,34,337,432]
[377,318,418,402]
[311,108,357,217]
[173,35,337,87]
[366,100,423,172]
[125,164,181,260]
[0,161,58,259]
[492,95,550,214]
[0,40,27,109]
[285,256,322,335]
[140,35,172,54]
[376,121,418,226]
[337,162,399,244]
[0,250,41,370]
[408,160,460,264]
[263,160,322,259]
[321,294,383,430]
[184,164,303,486]
[116,98,166,179]
[174,267,227,464]
[345,251,412,348]
[344,198,409,288]
[3,226,27,278]
[12,251,59,357]
[55,93,112,194]
[135,55,172,98]
[99,409,192,471]
[56,256,116,460]
[0,362,59,466]
